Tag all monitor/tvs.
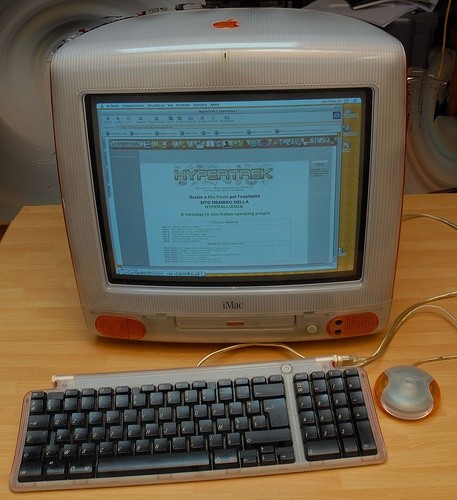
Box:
[51,7,408,341]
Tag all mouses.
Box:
[374,366,441,421]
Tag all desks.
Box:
[0,194,457,500]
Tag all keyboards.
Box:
[9,354,387,492]
